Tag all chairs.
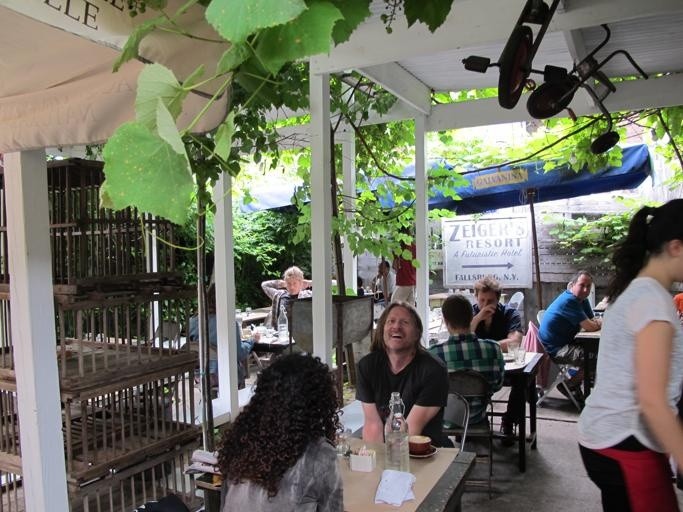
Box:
[523,319,581,412]
[537,307,547,326]
[441,287,543,452]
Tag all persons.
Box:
[426,295,506,443]
[470,277,523,447]
[576,198,683,512]
[538,271,602,407]
[355,303,455,447]
[213,353,344,512]
[187,226,416,389]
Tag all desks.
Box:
[325,437,479,507]
[573,327,601,399]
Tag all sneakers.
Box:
[503,412,515,435]
[557,380,585,406]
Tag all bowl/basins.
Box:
[410,436,431,453]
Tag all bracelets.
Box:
[476,316,481,320]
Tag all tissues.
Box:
[350,445,377,474]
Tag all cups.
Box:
[514,348,524,364]
[507,334,522,357]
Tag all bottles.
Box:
[277,305,287,341]
[385,392,409,474]
[235,307,242,338]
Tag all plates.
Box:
[502,353,514,362]
[409,445,437,458]
